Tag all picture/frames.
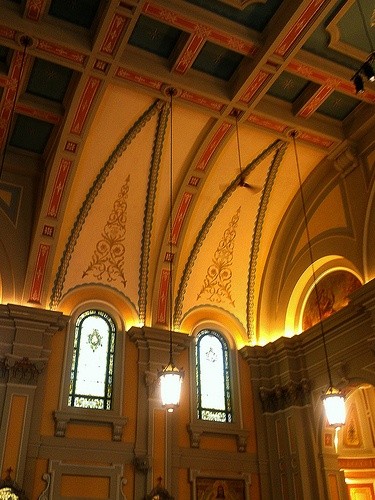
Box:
[195,476,247,500]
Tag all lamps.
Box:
[286,128,348,431]
[157,86,184,415]
[350,50,375,96]
